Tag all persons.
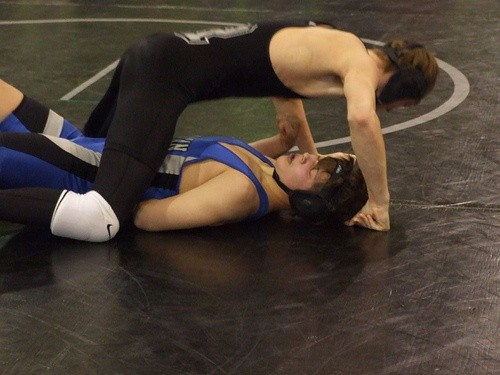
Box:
[0,77,370,239]
[0,20,440,245]
